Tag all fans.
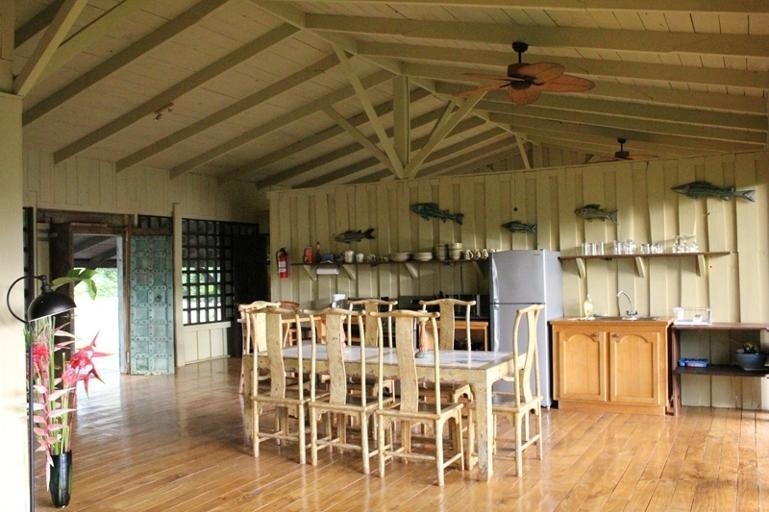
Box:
[449,41,595,106]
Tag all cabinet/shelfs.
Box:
[556,250,731,280]
[671,321,769,416]
[549,315,673,416]
[288,258,484,281]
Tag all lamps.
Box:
[7,274,77,511]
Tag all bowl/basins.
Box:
[736,353,768,370]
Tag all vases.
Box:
[46,450,72,508]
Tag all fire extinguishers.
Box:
[275,247,289,278]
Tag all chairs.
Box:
[237,301,282,352]
[300,307,393,475]
[347,299,399,441]
[369,310,464,486]
[418,298,476,450]
[465,304,544,477]
[242,307,330,465]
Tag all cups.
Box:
[585,243,594,255]
[464,250,473,260]
[640,243,649,254]
[488,249,564,411]
[473,248,481,260]
[481,248,488,259]
[577,243,585,256]
[671,234,684,253]
[613,239,637,255]
[650,241,663,254]
[684,233,697,252]
[592,241,605,255]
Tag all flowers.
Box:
[27,329,115,491]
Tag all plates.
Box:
[678,358,710,368]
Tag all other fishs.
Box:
[573,203,619,226]
[670,181,757,204]
[333,227,375,244]
[408,202,464,227]
[499,219,538,237]
